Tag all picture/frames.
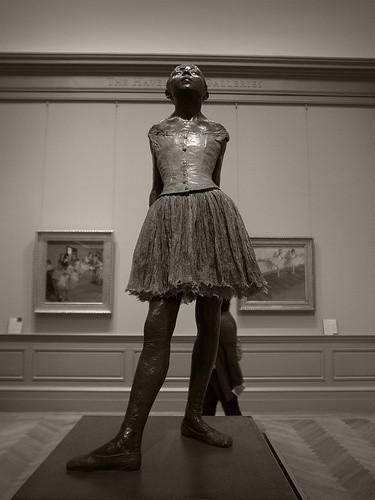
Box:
[236,236,316,312]
[34,230,115,315]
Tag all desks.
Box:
[11,416,297,499]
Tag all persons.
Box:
[201,298,246,416]
[65,62,268,475]
